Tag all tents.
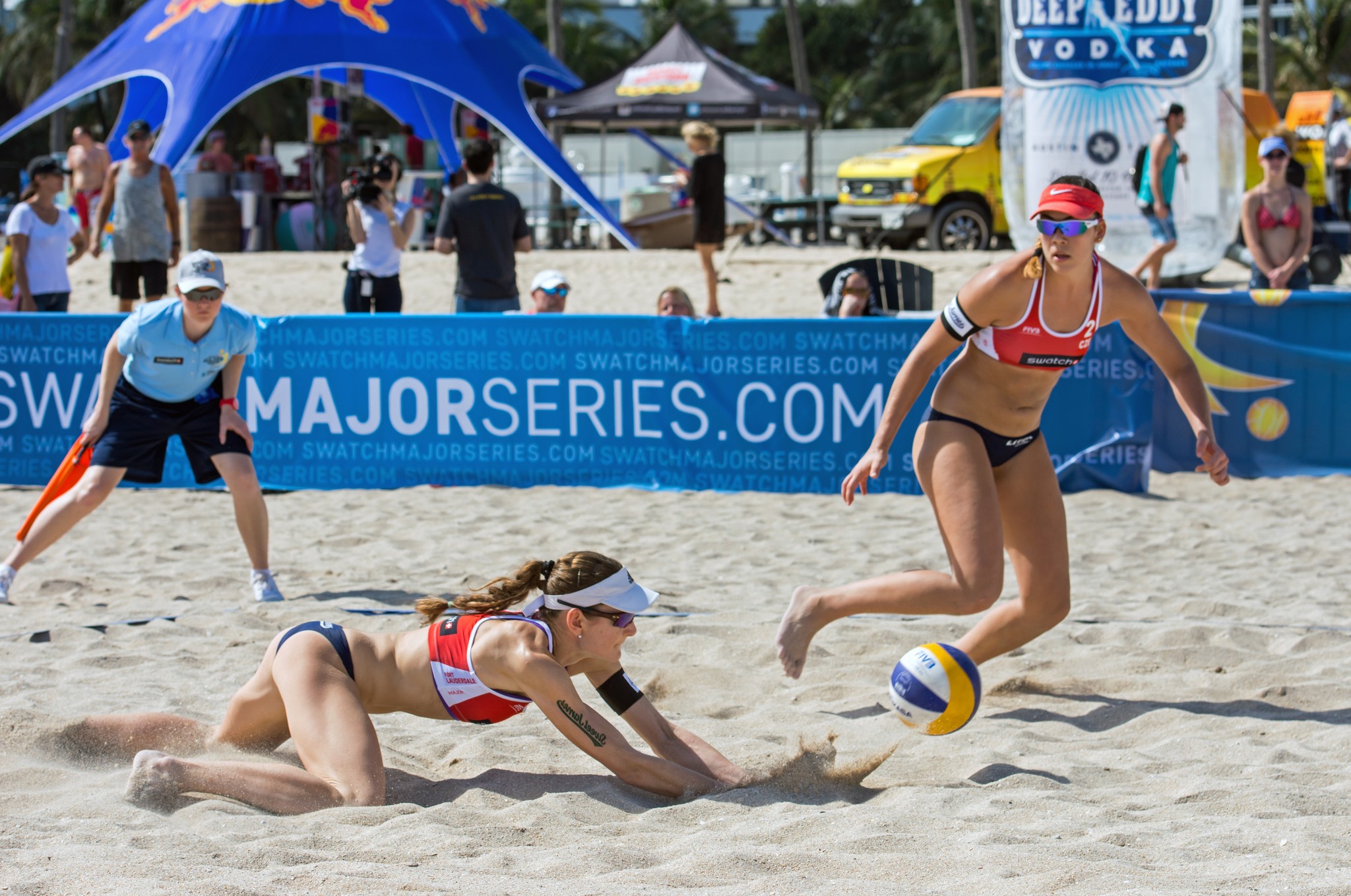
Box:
[531,18,825,249]
[0,2,811,252]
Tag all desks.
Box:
[265,190,314,251]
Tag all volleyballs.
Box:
[888,643,982,736]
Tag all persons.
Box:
[673,120,725,318]
[434,138,531,314]
[1130,102,1188,289]
[89,122,182,314]
[1240,110,1351,291]
[775,175,1230,683]
[0,246,285,605]
[62,124,424,251]
[822,268,887,318]
[658,287,695,319]
[504,267,572,314]
[27,550,749,817]
[6,156,87,313]
[339,153,416,315]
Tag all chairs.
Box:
[819,257,936,314]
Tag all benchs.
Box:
[761,195,846,246]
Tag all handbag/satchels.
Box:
[1133,147,1145,193]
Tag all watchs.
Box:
[219,397,240,410]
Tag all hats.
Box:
[1029,183,1104,221]
[176,249,225,293]
[531,270,570,292]
[1259,136,1288,157]
[1155,104,1183,121]
[520,565,658,618]
[127,120,149,138]
[27,159,73,181]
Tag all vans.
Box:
[1282,90,1351,226]
[828,87,1284,252]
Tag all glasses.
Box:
[556,598,637,629]
[842,286,868,297]
[542,287,567,297]
[1264,152,1286,160]
[1034,218,1104,237]
[129,134,148,141]
[184,284,228,302]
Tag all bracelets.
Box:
[388,218,398,228]
[170,239,181,246]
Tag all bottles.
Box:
[780,160,794,202]
